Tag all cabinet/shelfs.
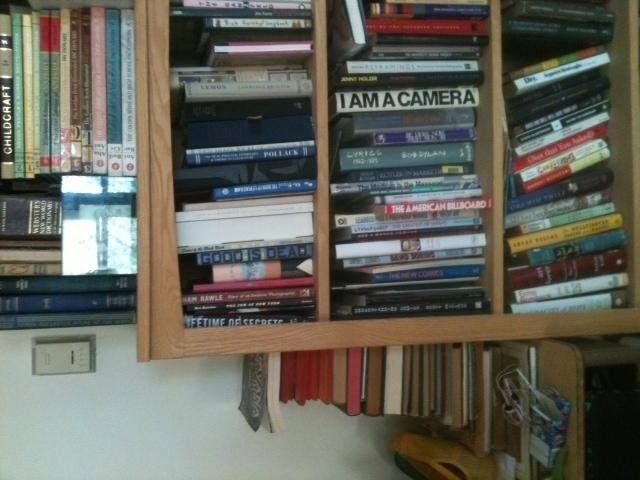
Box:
[136,0,640,363]
